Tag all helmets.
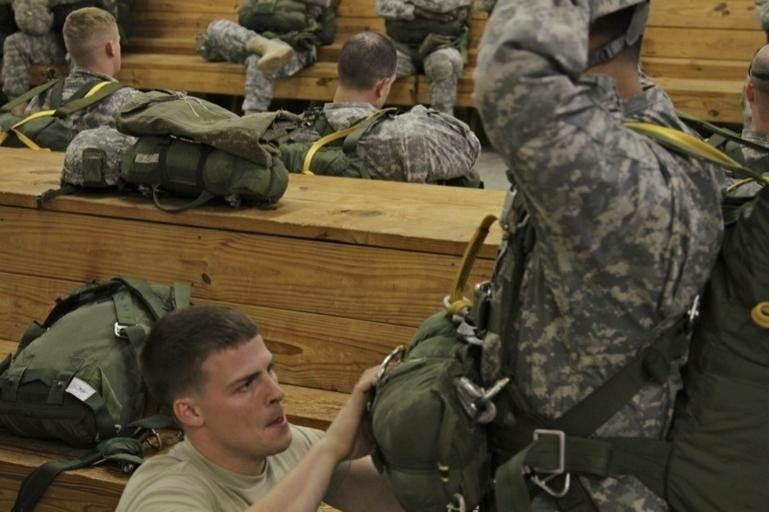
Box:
[60,125,137,189]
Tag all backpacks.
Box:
[0,76,128,151]
[623,108,769,511]
[115,92,306,208]
[0,274,194,446]
[280,107,399,176]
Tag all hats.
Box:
[477,0,650,24]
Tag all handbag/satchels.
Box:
[362,306,494,511]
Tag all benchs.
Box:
[0,145,511,512]
[115,0,768,125]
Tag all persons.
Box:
[275,30,483,183]
[702,42,769,183]
[194,1,343,114]
[111,303,405,512]
[741,0,769,126]
[374,0,476,116]
[0,1,71,103]
[471,0,728,512]
[23,5,144,152]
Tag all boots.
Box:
[247,33,302,71]
